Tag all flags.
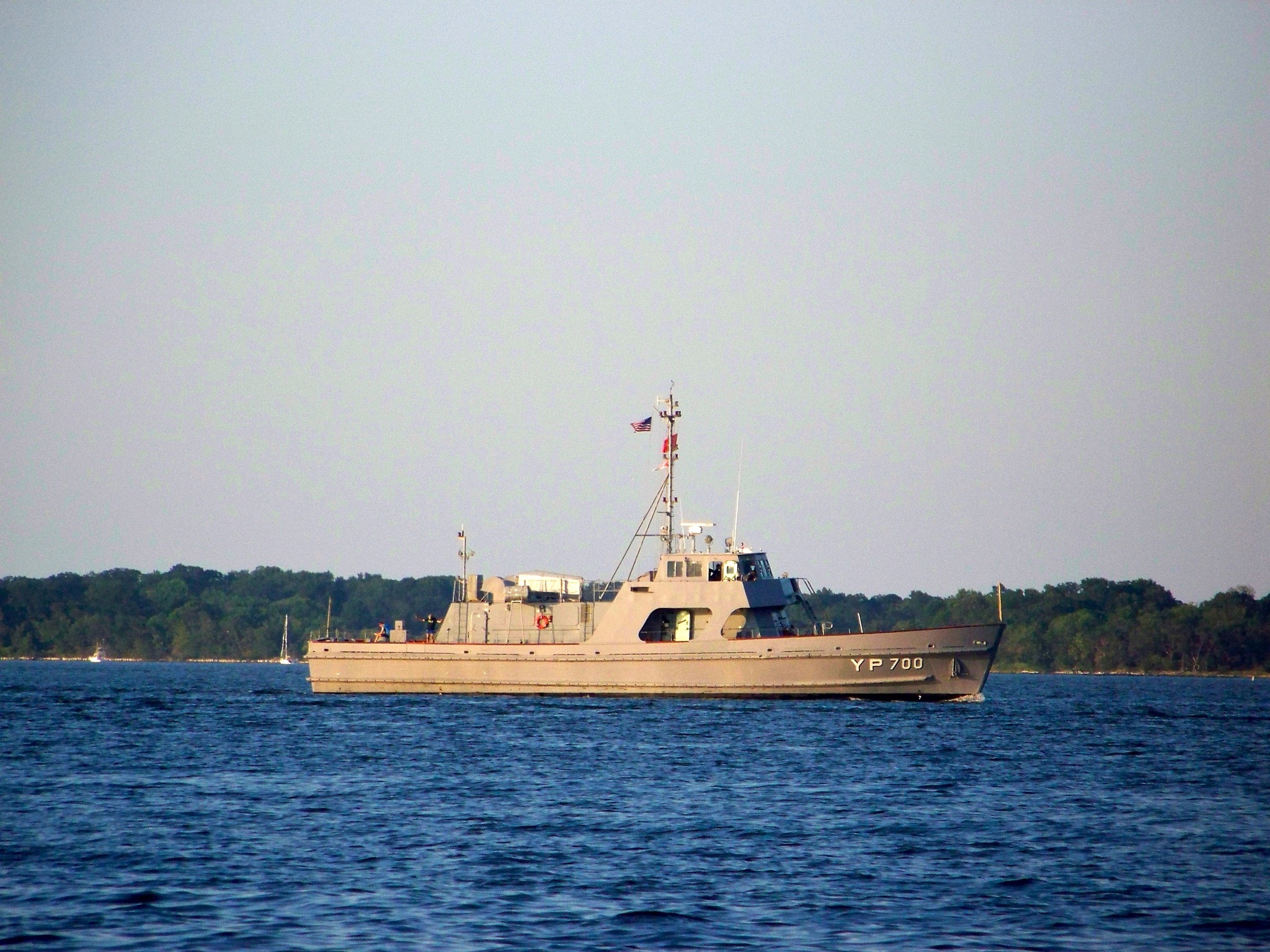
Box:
[630,417,652,432]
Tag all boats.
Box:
[301,378,1006,697]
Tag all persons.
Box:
[417,614,442,643]
[374,622,389,643]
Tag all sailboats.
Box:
[279,614,291,665]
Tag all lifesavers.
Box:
[536,615,549,629]
